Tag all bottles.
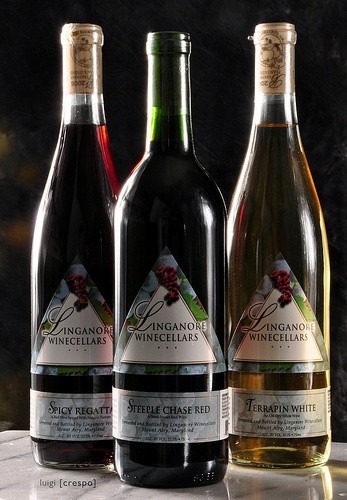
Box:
[227,22,333,470]
[111,30,229,489]
[28,22,123,470]
